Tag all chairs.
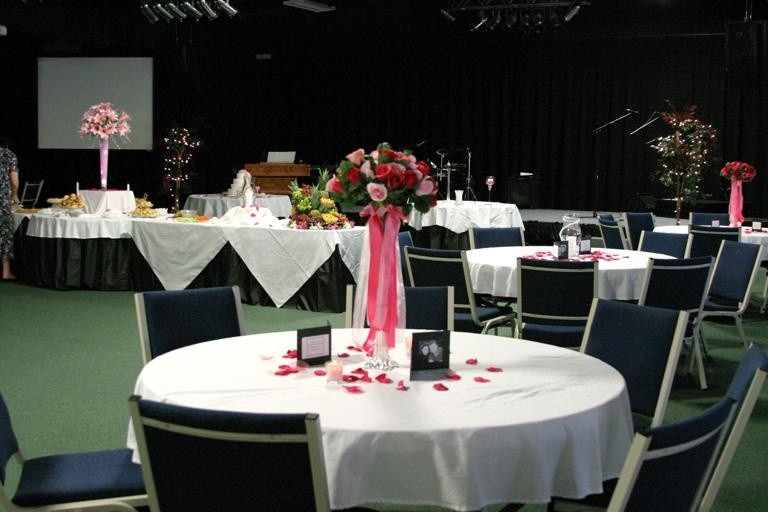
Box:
[689,224,742,261]
[637,256,713,390]
[497,297,690,510]
[683,239,764,389]
[1,395,151,512]
[403,246,515,340]
[696,342,767,512]
[467,225,526,250]
[514,257,600,353]
[598,214,616,221]
[688,211,731,226]
[597,218,624,249]
[127,393,331,511]
[606,396,737,512]
[132,285,246,366]
[394,231,411,279]
[621,213,655,251]
[636,229,695,261]
[14,180,45,209]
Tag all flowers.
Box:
[646,98,720,225]
[76,96,133,153]
[325,138,443,223]
[719,159,758,184]
[281,167,354,230]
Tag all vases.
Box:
[97,135,110,190]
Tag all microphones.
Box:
[626,108,639,114]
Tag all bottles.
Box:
[241,176,256,207]
[562,212,582,257]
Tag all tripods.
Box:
[569,131,613,222]
[427,145,478,201]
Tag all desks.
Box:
[78,188,135,214]
[404,198,524,247]
[129,324,638,511]
[6,207,376,313]
[463,245,653,307]
[182,192,290,217]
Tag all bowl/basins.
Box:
[47,204,169,217]
[181,209,197,217]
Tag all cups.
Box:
[405,336,412,354]
[325,361,343,387]
[455,190,464,205]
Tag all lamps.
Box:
[438,1,591,35]
[139,2,240,26]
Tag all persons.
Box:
[416,340,443,363]
[0,138,19,282]
